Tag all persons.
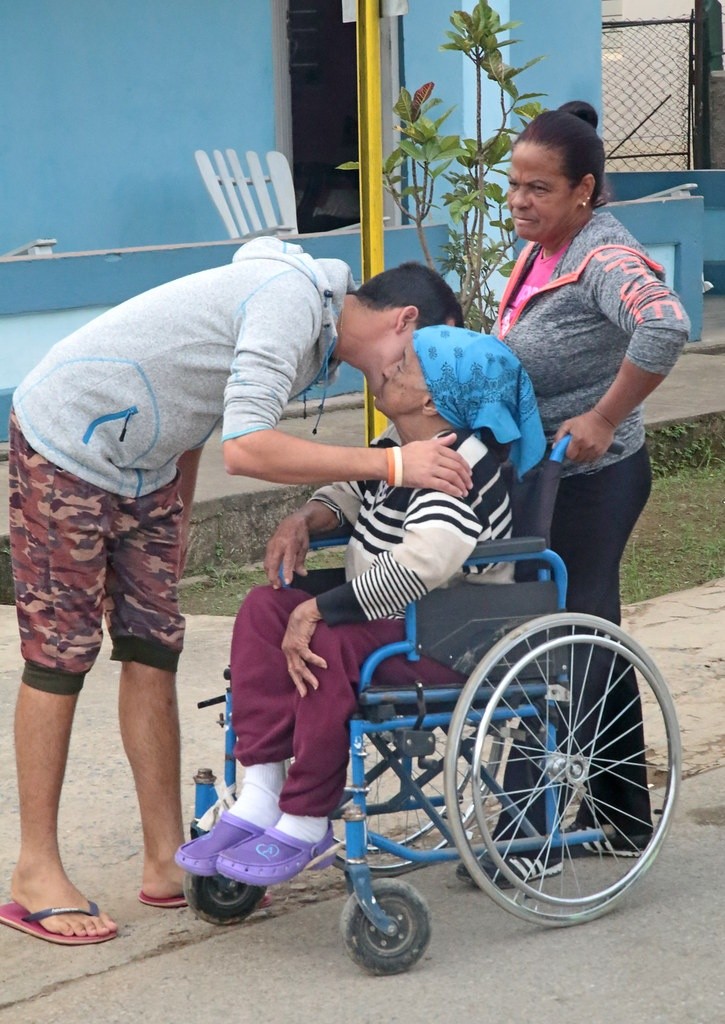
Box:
[173,324,548,886]
[0,237,473,946]
[452,99,693,888]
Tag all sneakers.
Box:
[557,822,653,858]
[455,849,562,888]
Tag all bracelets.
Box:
[387,446,403,488]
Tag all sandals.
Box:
[175,811,266,875]
[216,817,337,885]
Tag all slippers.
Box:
[136,887,272,908]
[0,896,116,945]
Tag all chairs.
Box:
[194,147,390,241]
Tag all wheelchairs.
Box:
[181,430,684,976]
